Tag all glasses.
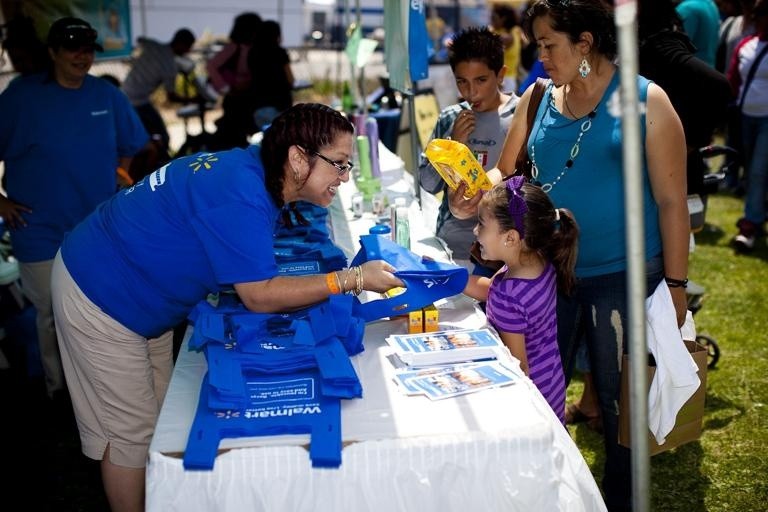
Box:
[311,152,354,176]
[545,0,571,26]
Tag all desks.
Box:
[144,131,609,508]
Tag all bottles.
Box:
[342,80,352,113]
[369,197,410,250]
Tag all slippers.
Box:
[563,405,596,425]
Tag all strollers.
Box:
[684,144,734,372]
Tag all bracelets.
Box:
[327,265,363,296]
[663,273,689,286]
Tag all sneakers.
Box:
[734,221,759,251]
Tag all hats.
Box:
[48,18,103,53]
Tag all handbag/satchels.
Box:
[469,76,553,271]
[618,335,708,456]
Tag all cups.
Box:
[371,194,383,216]
[352,194,363,217]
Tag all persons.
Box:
[420,176,578,428]
[441,0,690,512]
[49,102,408,512]
[0,1,768,397]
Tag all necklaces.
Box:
[531,83,601,194]
[563,84,584,121]
